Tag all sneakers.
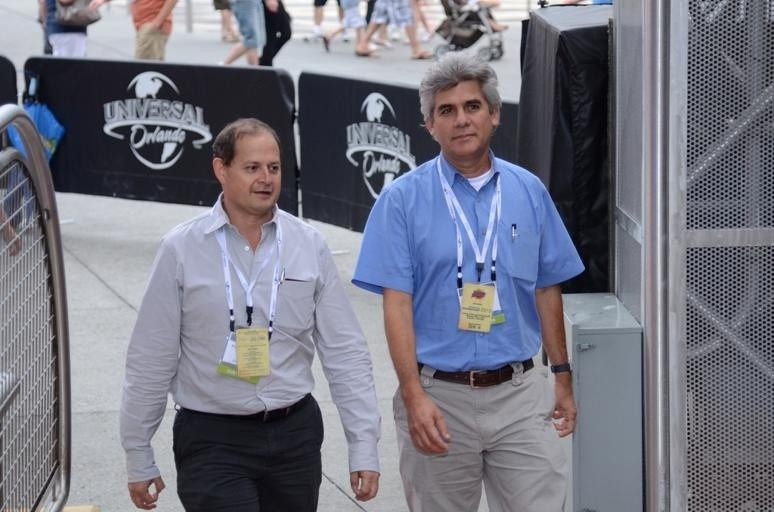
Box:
[308,32,434,60]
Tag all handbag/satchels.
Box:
[52,0,101,28]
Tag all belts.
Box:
[178,392,312,425]
[414,357,535,388]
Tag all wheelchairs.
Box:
[434,0,502,62]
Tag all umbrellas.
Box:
[7,72,65,228]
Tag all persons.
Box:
[119,116,385,511]
[0,205,23,257]
[129,0,177,60]
[37,0,113,57]
[350,52,587,511]
[213,0,509,67]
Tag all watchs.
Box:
[549,362,574,373]
